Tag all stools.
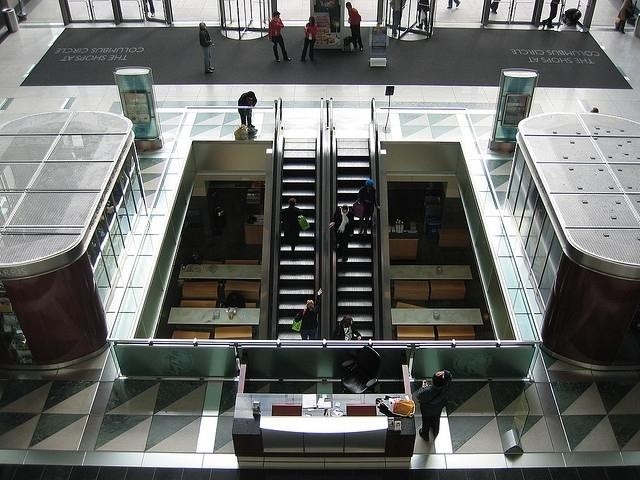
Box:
[167,218,261,339]
[391,227,487,341]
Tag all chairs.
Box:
[342,345,383,393]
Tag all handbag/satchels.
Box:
[353,200,365,219]
[297,215,310,231]
[394,400,415,417]
[234,124,248,141]
[292,318,302,332]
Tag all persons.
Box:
[445,0,462,9]
[356,178,375,236]
[490,0,501,15]
[298,15,315,62]
[329,204,354,261]
[389,0,407,37]
[541,0,559,28]
[294,300,317,340]
[346,1,363,51]
[417,369,453,441]
[614,0,634,34]
[237,90,257,128]
[333,314,361,340]
[199,22,215,74]
[279,197,300,250]
[268,10,292,63]
[414,0,429,31]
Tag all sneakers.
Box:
[419,428,429,441]
